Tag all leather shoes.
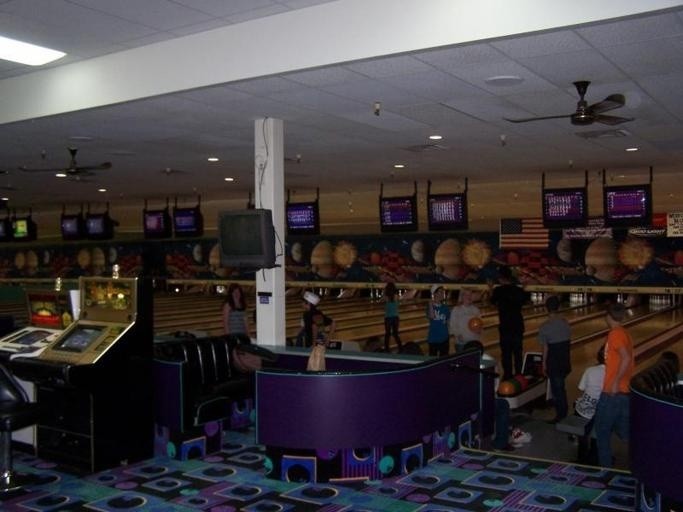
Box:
[547,418,560,424]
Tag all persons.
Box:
[223,282,250,335]
[451,289,482,353]
[540,295,571,424]
[377,282,403,354]
[596,303,634,475]
[487,268,532,379]
[427,282,451,357]
[575,344,607,421]
[298,292,335,349]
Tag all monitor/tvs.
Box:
[379,196,417,232]
[541,186,589,229]
[11,329,53,347]
[10,218,36,242]
[173,208,204,238]
[286,202,320,236]
[142,208,172,239]
[60,212,83,240]
[51,324,109,354]
[602,183,653,226]
[82,213,115,241]
[426,193,468,231]
[219,208,276,269]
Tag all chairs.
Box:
[0,364,95,499]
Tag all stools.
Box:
[556,413,613,466]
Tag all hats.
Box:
[303,291,321,305]
[430,283,444,295]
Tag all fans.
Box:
[17,148,112,175]
[502,80,635,126]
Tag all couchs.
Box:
[171,334,276,457]
[630,358,682,501]
